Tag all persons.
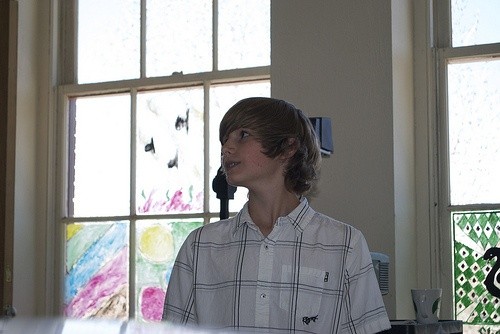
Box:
[161,97,392,334]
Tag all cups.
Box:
[410,288,443,324]
